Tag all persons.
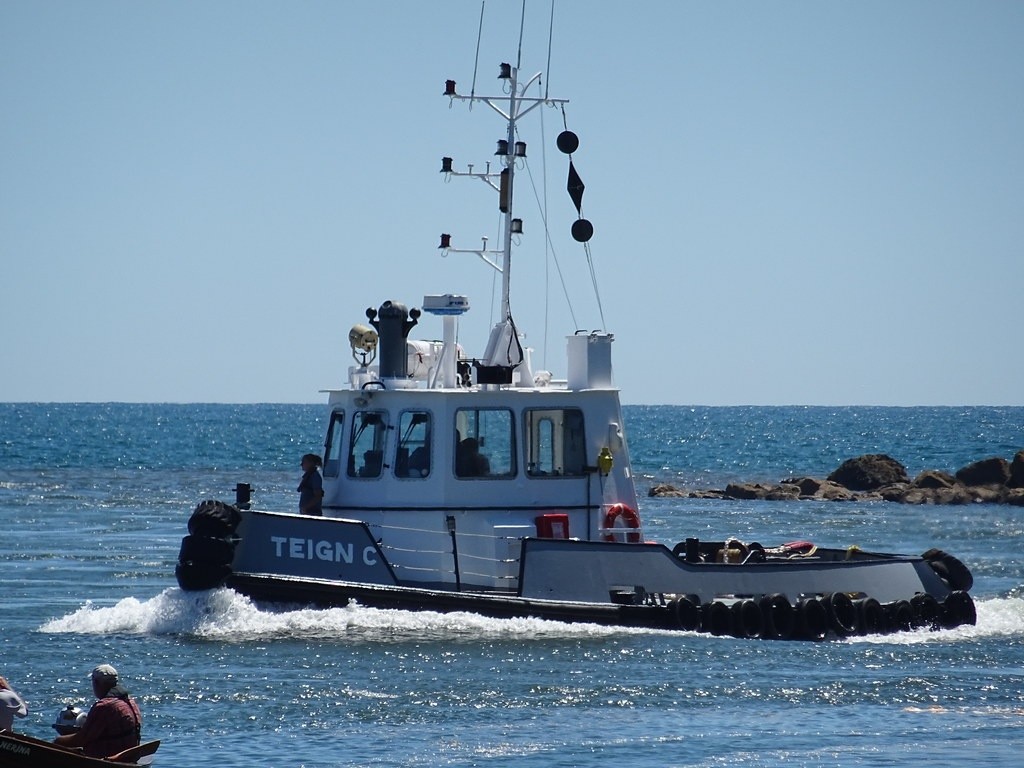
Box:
[296,454,325,516]
[51,663,143,761]
[0,676,29,733]
[455,429,477,477]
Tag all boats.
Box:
[0,728,161,768]
[175,0,980,638]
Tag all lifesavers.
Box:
[668,589,978,644]
[603,502,642,543]
